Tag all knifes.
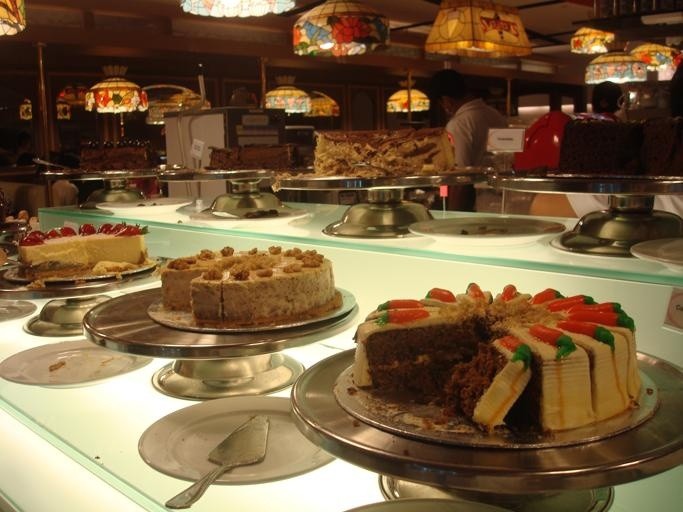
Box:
[165,413,268,507]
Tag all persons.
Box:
[425,69,512,171]
[567,81,627,123]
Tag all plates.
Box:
[191,210,311,223]
[630,241,683,271]
[96,200,193,214]
[1,339,155,388]
[139,393,339,484]
[0,300,36,322]
[409,218,566,240]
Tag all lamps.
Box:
[147,92,211,125]
[181,0,295,18]
[56,87,87,119]
[85,77,148,114]
[262,85,312,114]
[0,0,25,35]
[305,90,340,116]
[585,52,647,84]
[658,50,683,81]
[628,43,679,70]
[20,98,31,119]
[424,0,532,58]
[387,89,430,112]
[292,0,390,55]
[571,27,624,54]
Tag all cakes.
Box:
[204,141,295,174]
[558,118,682,180]
[313,128,456,176]
[79,146,149,173]
[351,283,645,440]
[158,244,345,327]
[15,223,152,286]
